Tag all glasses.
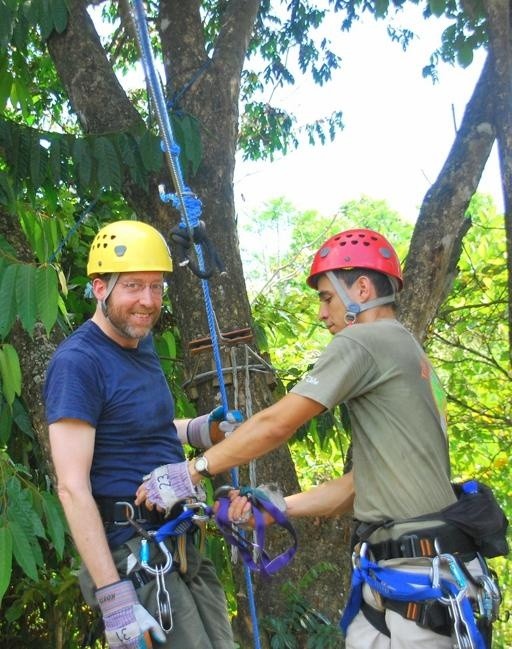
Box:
[113,279,165,296]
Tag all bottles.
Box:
[461,481,482,499]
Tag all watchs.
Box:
[188,454,212,479]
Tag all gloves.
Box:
[93,576,170,649]
[184,403,244,452]
[140,457,199,517]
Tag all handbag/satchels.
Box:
[437,476,509,558]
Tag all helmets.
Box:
[304,226,406,293]
[85,220,176,276]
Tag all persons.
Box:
[41,215,244,649]
[136,227,491,646]
